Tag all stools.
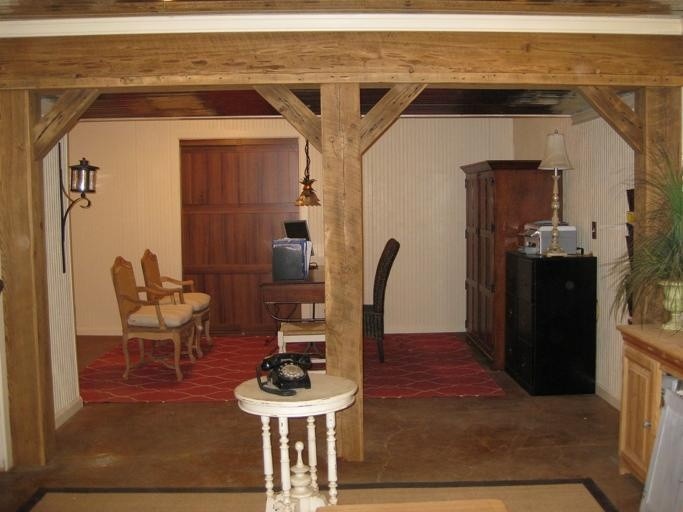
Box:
[276,320,327,369]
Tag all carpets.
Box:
[78,330,505,404]
[13,474,619,512]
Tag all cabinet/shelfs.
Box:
[458,155,564,370]
[504,245,598,397]
[616,314,683,488]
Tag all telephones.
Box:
[260,351,311,391]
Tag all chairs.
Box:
[140,249,213,359]
[108,256,195,381]
[363,239,400,362]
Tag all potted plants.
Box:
[597,132,683,333]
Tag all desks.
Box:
[233,372,360,512]
[256,263,326,360]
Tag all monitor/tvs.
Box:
[282,219,315,269]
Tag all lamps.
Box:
[57,139,100,275]
[294,138,321,209]
[537,128,574,255]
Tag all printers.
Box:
[516,220,577,255]
